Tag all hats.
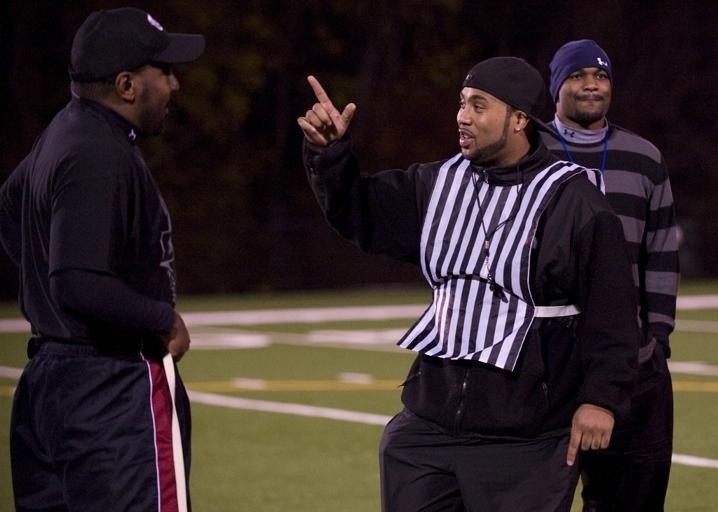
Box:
[64,3,208,81]
[459,55,561,140]
[545,36,616,104]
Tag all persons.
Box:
[2,6,210,510]
[297,58,642,512]
[541,40,686,510]
